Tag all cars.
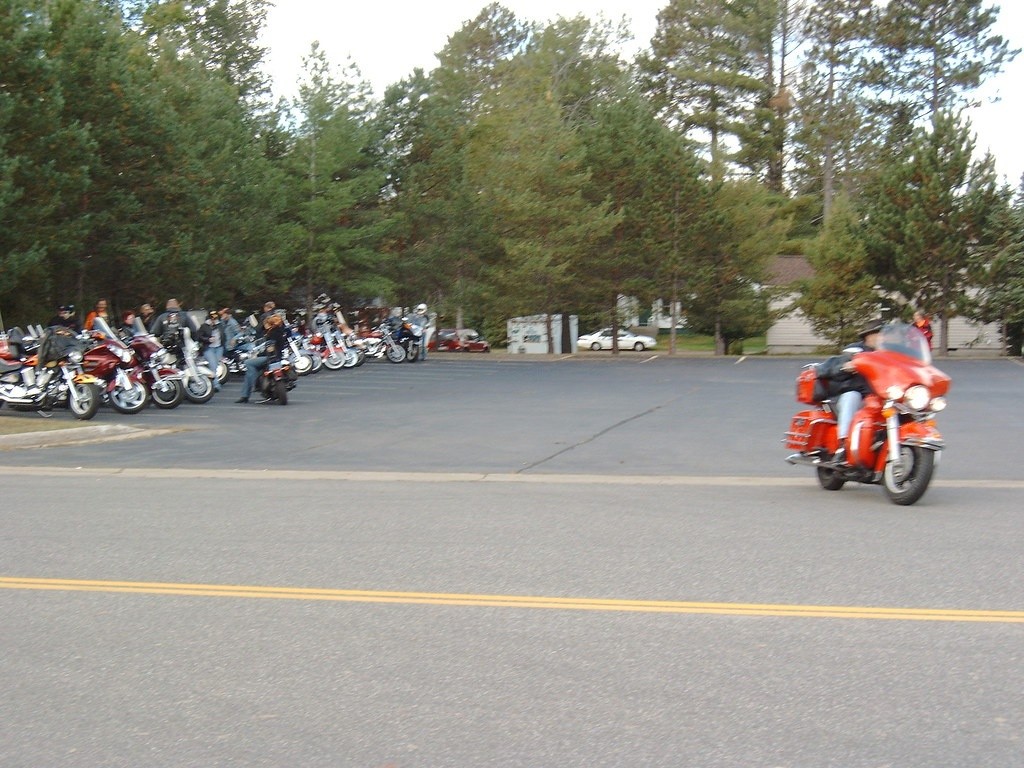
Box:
[577,327,657,352]
[428,329,492,354]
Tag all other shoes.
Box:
[834,445,844,461]
[234,398,248,403]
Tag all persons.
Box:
[198,308,243,393]
[37,298,79,418]
[401,303,428,361]
[254,302,288,355]
[118,303,157,346]
[232,315,284,404]
[150,299,197,351]
[290,304,339,339]
[82,299,109,333]
[904,311,933,353]
[814,320,889,462]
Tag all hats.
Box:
[858,320,886,337]
[209,309,218,318]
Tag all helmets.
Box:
[416,303,427,315]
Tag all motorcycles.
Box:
[0,309,428,421]
[781,321,951,505]
